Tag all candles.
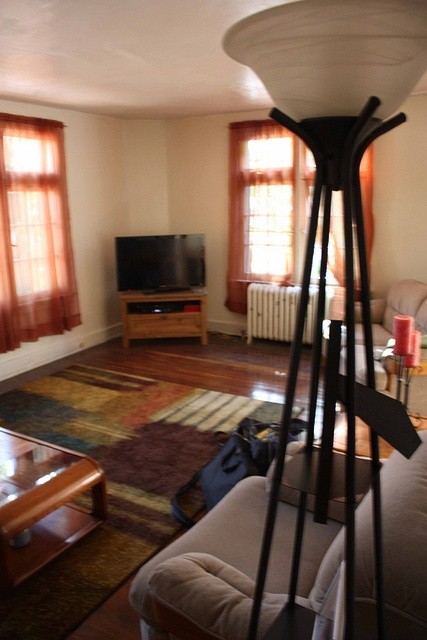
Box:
[403,331,420,367]
[392,314,413,355]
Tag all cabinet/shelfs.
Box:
[118,291,207,347]
[315,412,427,459]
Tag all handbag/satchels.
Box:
[198,417,308,510]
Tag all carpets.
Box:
[0,362,305,639]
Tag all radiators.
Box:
[246,282,333,356]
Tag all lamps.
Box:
[222,1,427,639]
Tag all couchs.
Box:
[127,430,427,639]
[354,279,427,416]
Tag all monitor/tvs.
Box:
[115,233,206,295]
